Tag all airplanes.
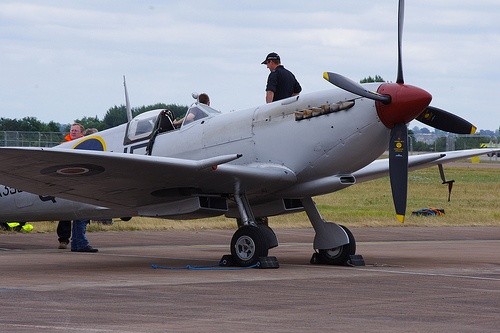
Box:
[0,0,500,268]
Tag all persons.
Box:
[173,93,210,125]
[261,53,302,104]
[57,124,101,253]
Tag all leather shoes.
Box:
[71,240,98,253]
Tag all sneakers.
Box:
[58,240,67,249]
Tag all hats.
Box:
[260,52,281,64]
